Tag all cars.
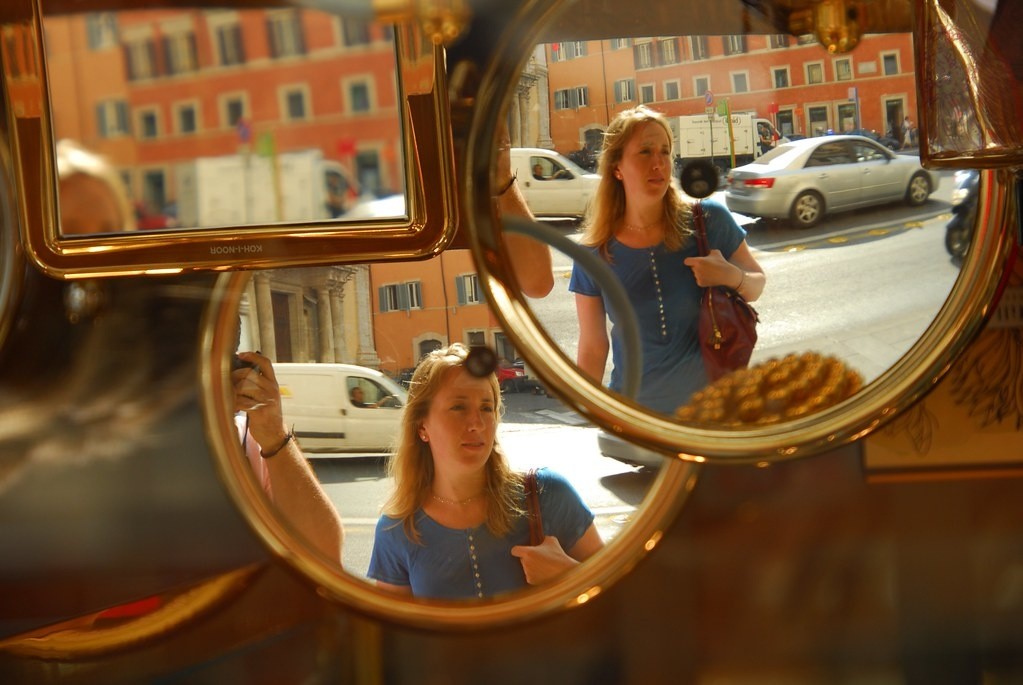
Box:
[496,358,546,396]
[724,132,942,229]
[509,146,604,220]
[843,127,901,152]
[784,134,805,142]
[597,426,663,473]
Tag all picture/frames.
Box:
[861,262,1023,484]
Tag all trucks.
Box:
[169,145,380,230]
[664,109,792,190]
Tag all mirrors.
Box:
[0,0,1020,637]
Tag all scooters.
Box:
[944,166,983,258]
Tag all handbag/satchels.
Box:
[692,196,760,380]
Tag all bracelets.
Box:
[735,272,745,292]
[494,176,516,198]
[260,434,292,458]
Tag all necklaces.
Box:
[623,218,663,232]
[432,493,484,505]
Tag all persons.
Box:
[760,133,771,154]
[367,343,604,600]
[901,116,913,151]
[56,138,136,234]
[491,113,555,298]
[568,105,766,418]
[533,165,566,181]
[232,312,346,561]
[350,387,392,408]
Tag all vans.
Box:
[268,362,415,462]
